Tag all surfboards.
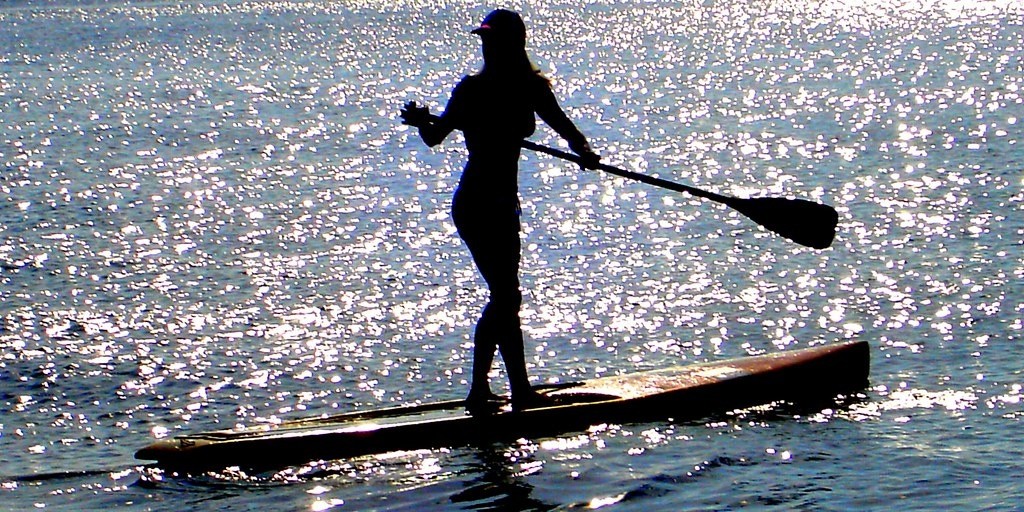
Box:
[134,337,869,466]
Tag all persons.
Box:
[400,9,602,410]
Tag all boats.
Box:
[132,339,874,475]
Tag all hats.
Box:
[470,9,526,37]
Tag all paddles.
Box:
[400,101,840,251]
